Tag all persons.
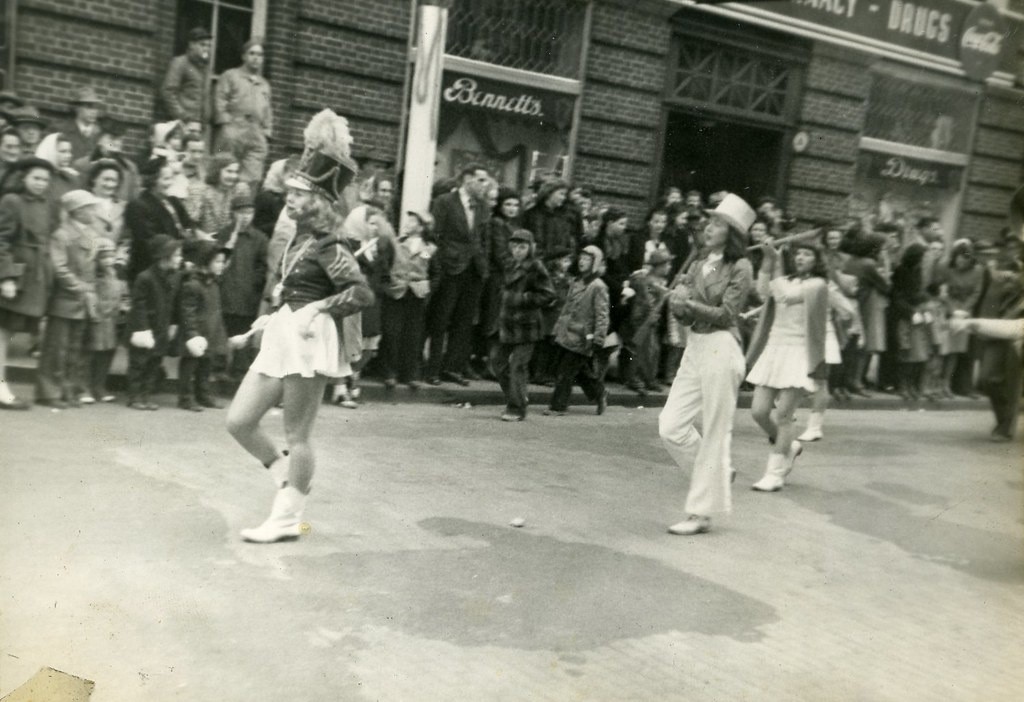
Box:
[744,240,828,493]
[223,148,375,543]
[979,256,1024,441]
[0,87,1024,421]
[213,39,273,198]
[658,193,757,535]
[947,317,1024,343]
[771,236,854,442]
[160,25,213,139]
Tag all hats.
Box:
[93,239,117,251]
[509,229,606,274]
[19,158,54,174]
[644,240,675,264]
[68,87,102,102]
[187,27,212,41]
[59,189,105,213]
[145,233,186,259]
[230,196,257,210]
[240,38,267,54]
[285,108,357,204]
[0,91,52,130]
[703,193,758,239]
[407,208,436,231]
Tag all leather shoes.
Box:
[0,396,29,411]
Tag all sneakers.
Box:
[668,514,710,536]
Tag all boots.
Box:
[331,385,361,408]
[80,349,116,404]
[752,436,803,491]
[798,412,825,441]
[240,450,312,544]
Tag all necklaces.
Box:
[271,233,318,299]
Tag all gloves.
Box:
[132,329,156,349]
[186,336,208,358]
[295,304,320,341]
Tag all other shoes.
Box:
[384,373,480,386]
[177,398,226,414]
[501,395,529,421]
[597,389,610,415]
[130,402,160,410]
[40,397,80,408]
[832,386,979,401]
[543,407,569,417]
